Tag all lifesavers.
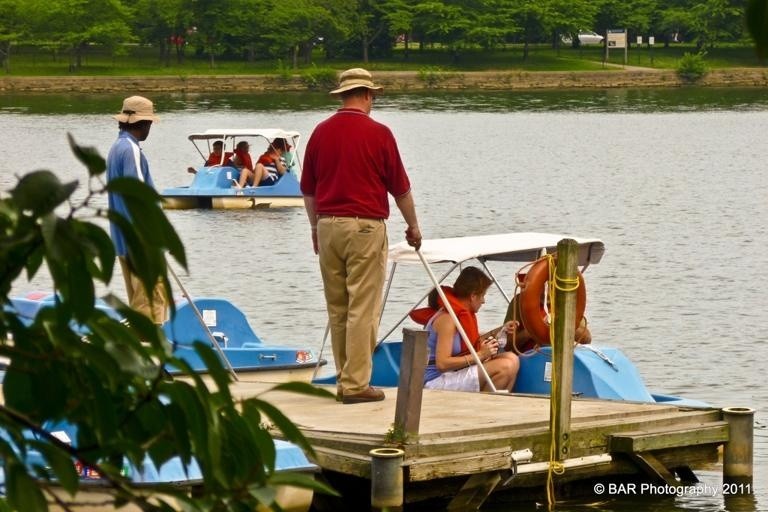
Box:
[518,256,587,347]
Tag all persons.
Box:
[188,138,292,189]
[107,95,170,325]
[300,68,422,404]
[423,266,520,392]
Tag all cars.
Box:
[557,27,607,45]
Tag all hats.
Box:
[113,95,161,124]
[329,67,384,96]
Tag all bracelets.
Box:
[464,355,471,367]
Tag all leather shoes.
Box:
[336,387,384,404]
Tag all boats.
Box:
[311,232,713,410]
[2,290,331,387]
[0,366,320,508]
[157,126,311,211]
